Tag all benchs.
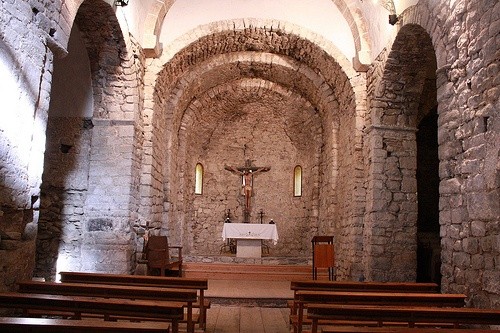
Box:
[0,272,210,333]
[286,280,500,332]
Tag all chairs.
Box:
[146,236,183,278]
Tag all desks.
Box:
[222,223,279,258]
